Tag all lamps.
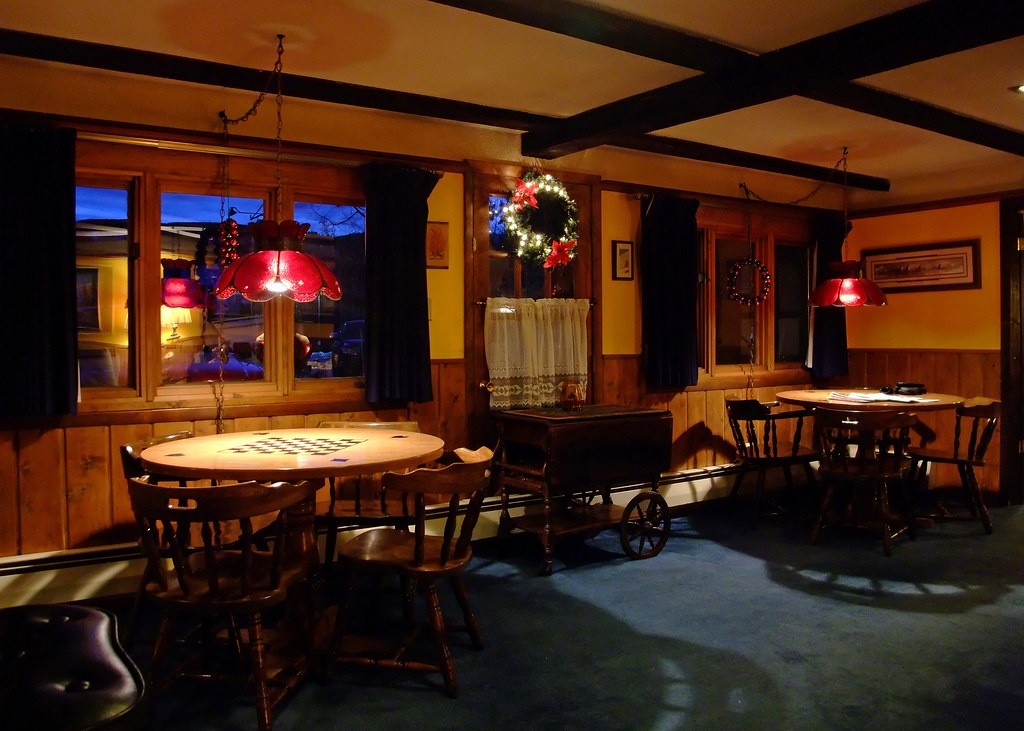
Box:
[807,146,887,307]
[161,258,215,309]
[213,34,343,304]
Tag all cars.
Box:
[328,318,366,377]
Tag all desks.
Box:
[141,427,445,677]
[495,403,673,576]
[777,389,966,530]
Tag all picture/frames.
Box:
[860,238,982,293]
[612,240,634,281]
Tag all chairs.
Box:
[0,603,150,731]
[119,421,490,730]
[723,383,1004,556]
